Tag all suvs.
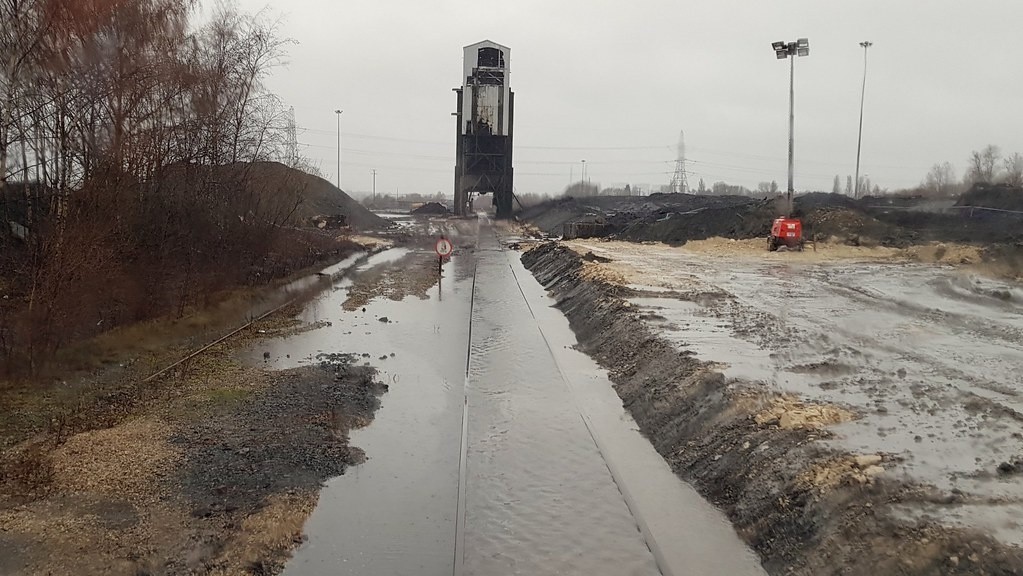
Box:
[766,218,805,251]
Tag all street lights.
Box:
[334,109,344,189]
[854,41,874,201]
[772,38,811,217]
